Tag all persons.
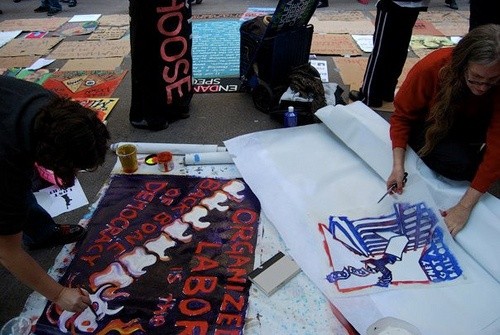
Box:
[0,77,110,312]
[349,0,500,109]
[387,24,500,238]
[34,0,77,16]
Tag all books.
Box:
[247,252,301,297]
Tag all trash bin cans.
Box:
[239,18,315,98]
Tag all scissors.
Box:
[378,172,409,204]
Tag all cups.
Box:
[116,144,138,174]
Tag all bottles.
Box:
[284,107,297,127]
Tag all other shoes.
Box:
[47,9,62,16]
[68,0,77,6]
[25,224,86,250]
[349,89,361,101]
[131,118,169,130]
[34,6,51,13]
[178,107,191,119]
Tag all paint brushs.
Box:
[73,281,100,319]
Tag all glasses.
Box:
[468,65,498,87]
